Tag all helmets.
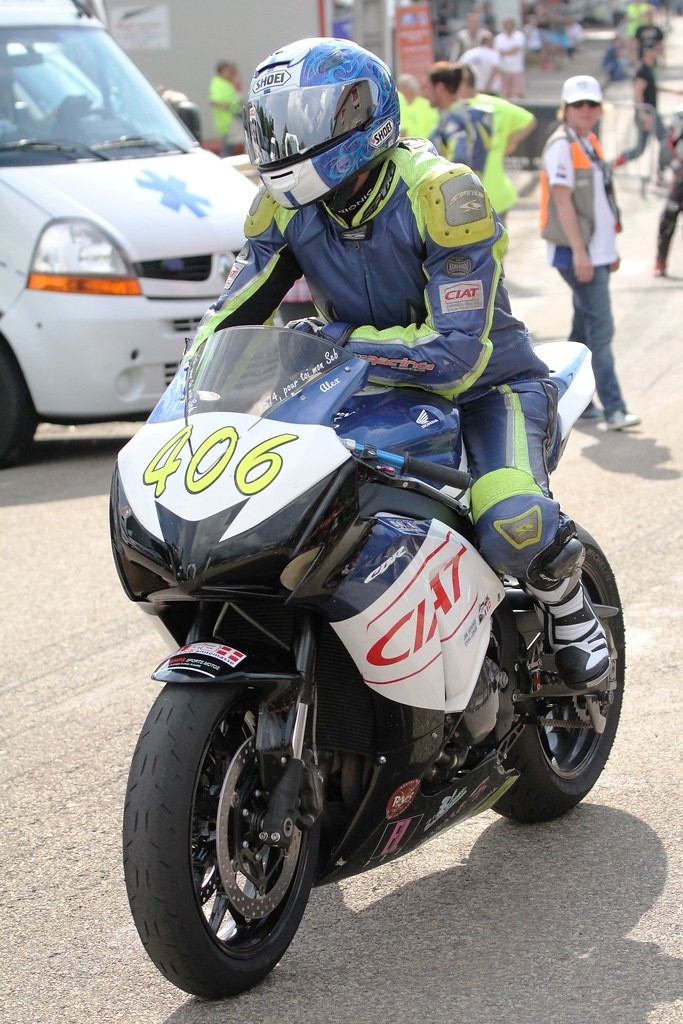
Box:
[246,37,401,210]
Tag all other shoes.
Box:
[580,408,603,418]
[654,259,666,276]
[606,409,640,430]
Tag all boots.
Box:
[518,519,614,691]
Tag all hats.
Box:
[562,75,603,104]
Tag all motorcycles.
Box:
[104,321,627,998]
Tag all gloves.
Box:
[279,316,356,371]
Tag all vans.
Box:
[0,1,261,465]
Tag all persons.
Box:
[655,112,682,276]
[396,2,683,183]
[541,75,640,432]
[161,61,247,158]
[181,36,613,689]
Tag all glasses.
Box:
[571,101,600,108]
